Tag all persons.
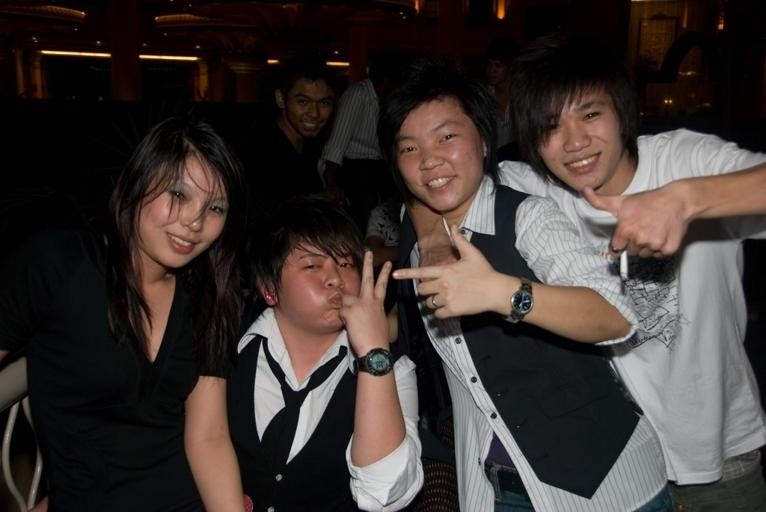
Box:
[227,194,429,510]
[0,113,249,511]
[231,41,516,268]
[377,54,675,511]
[490,21,766,510]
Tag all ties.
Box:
[260,338,348,466]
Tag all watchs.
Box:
[506,275,535,323]
[353,346,394,375]
